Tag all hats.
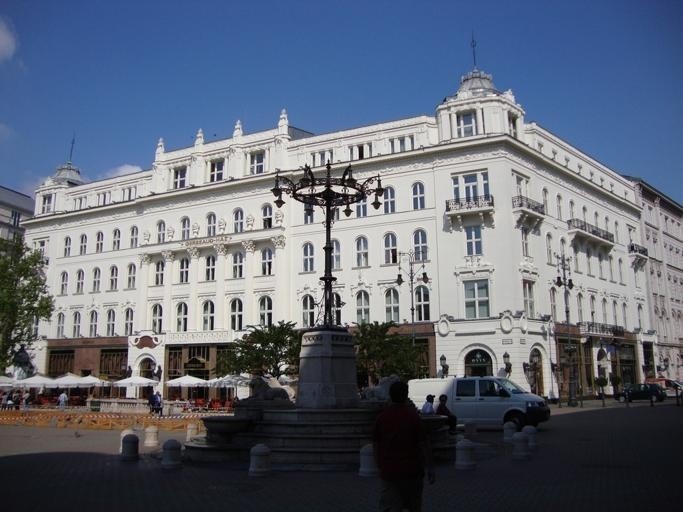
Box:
[426,394,437,400]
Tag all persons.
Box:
[203,401,211,413]
[437,394,459,435]
[0,389,30,411]
[186,400,195,412]
[421,394,436,414]
[147,390,163,416]
[372,380,436,511]
[58,391,68,410]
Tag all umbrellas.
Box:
[0,371,251,399]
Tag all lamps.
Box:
[120,362,132,377]
[502,351,512,372]
[149,360,162,382]
[440,354,448,374]
[550,352,566,372]
[522,352,540,372]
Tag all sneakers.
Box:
[448,429,457,435]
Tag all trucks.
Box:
[404,375,550,434]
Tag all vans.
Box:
[644,377,683,400]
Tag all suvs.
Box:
[613,383,665,403]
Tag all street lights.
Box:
[395,248,430,346]
[553,249,578,407]
